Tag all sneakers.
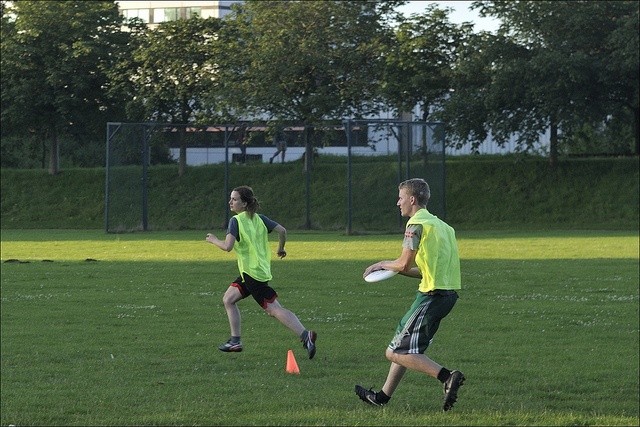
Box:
[218,339,243,352]
[302,329,317,359]
[443,369,465,411]
[354,383,383,408]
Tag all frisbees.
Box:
[364,270,399,283]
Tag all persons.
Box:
[353,176,465,411]
[204,186,317,359]
